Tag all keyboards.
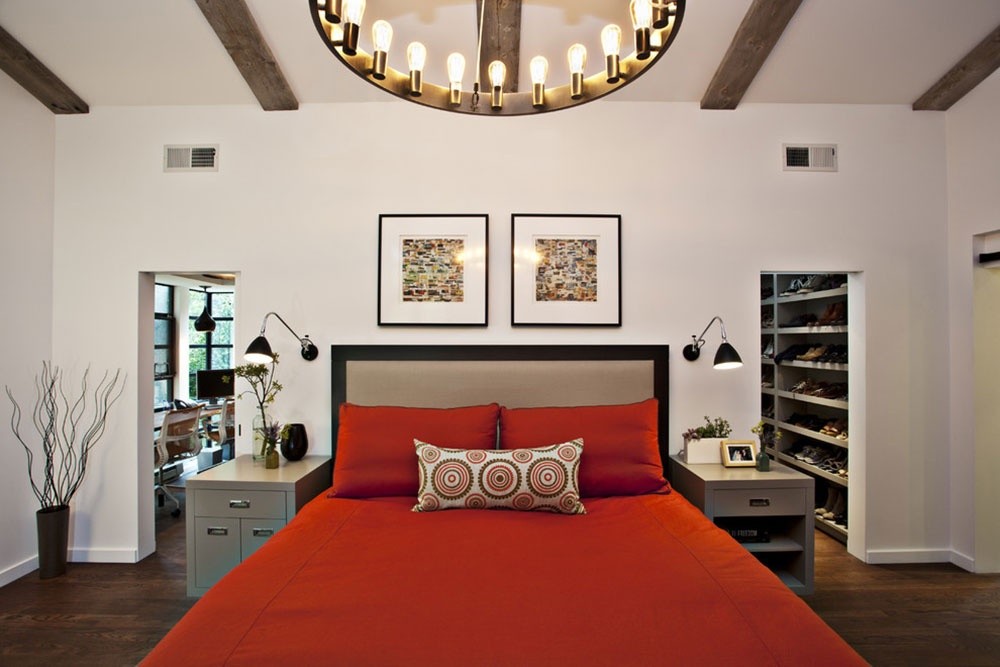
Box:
[205,405,222,409]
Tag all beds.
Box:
[131,342,883,667]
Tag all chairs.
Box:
[201,397,235,460]
[153,403,206,518]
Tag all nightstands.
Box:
[666,451,817,597]
[183,454,334,600]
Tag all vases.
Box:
[265,447,280,471]
[683,437,730,465]
[280,422,310,463]
[36,505,71,579]
[755,447,774,473]
[250,404,275,468]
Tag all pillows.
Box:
[325,402,501,497]
[408,434,590,518]
[508,396,674,500]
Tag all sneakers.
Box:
[805,447,829,464]
[794,447,816,461]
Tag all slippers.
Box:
[827,453,849,472]
[819,447,843,469]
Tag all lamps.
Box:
[680,315,745,374]
[241,310,320,368]
[307,0,690,119]
[193,284,217,334]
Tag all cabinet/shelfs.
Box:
[758,267,852,547]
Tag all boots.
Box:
[822,492,847,519]
[815,487,840,514]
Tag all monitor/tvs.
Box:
[196,370,234,403]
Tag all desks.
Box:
[153,404,225,449]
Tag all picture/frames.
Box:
[509,212,623,330]
[376,212,491,329]
[719,438,759,469]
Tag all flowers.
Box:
[258,421,284,456]
[750,419,784,453]
[680,414,734,442]
[219,353,293,457]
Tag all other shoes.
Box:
[783,442,807,456]
[834,514,847,525]
[760,275,848,441]
